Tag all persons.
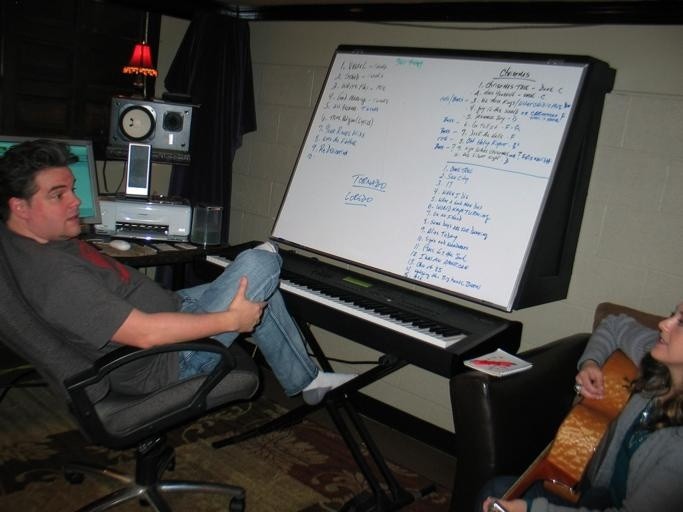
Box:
[480,300,683,512]
[0,139,360,407]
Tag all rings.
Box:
[573,382,582,396]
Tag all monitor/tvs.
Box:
[0,135,102,225]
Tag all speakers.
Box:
[108,97,192,151]
[126,143,150,193]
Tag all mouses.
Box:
[107,238,132,251]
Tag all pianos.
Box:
[195,241,524,380]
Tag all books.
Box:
[463,347,535,377]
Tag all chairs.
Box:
[0,237,252,512]
[447,302,671,510]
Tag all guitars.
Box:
[487,350,639,512]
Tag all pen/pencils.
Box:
[473,360,516,367]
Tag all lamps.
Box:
[123,43,157,98]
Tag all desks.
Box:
[83,236,232,267]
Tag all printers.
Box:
[95,193,190,242]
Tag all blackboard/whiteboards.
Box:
[268,48,590,313]
